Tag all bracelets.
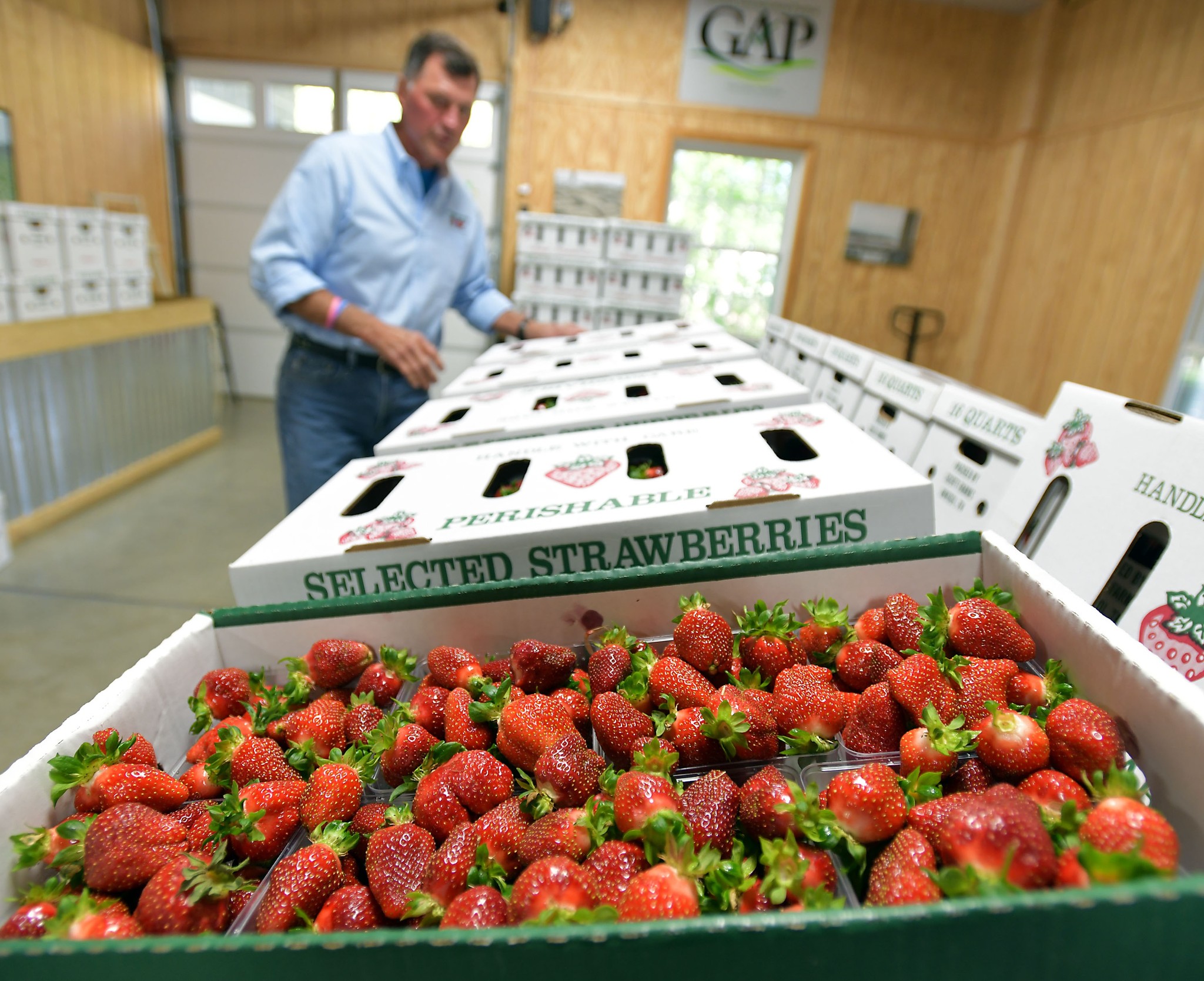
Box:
[516,318,532,339]
[325,295,348,329]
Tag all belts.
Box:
[290,333,394,374]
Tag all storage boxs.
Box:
[0,213,1204,981]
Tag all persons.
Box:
[251,29,588,516]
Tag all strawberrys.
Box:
[497,458,664,497]
[0,576,1178,939]
